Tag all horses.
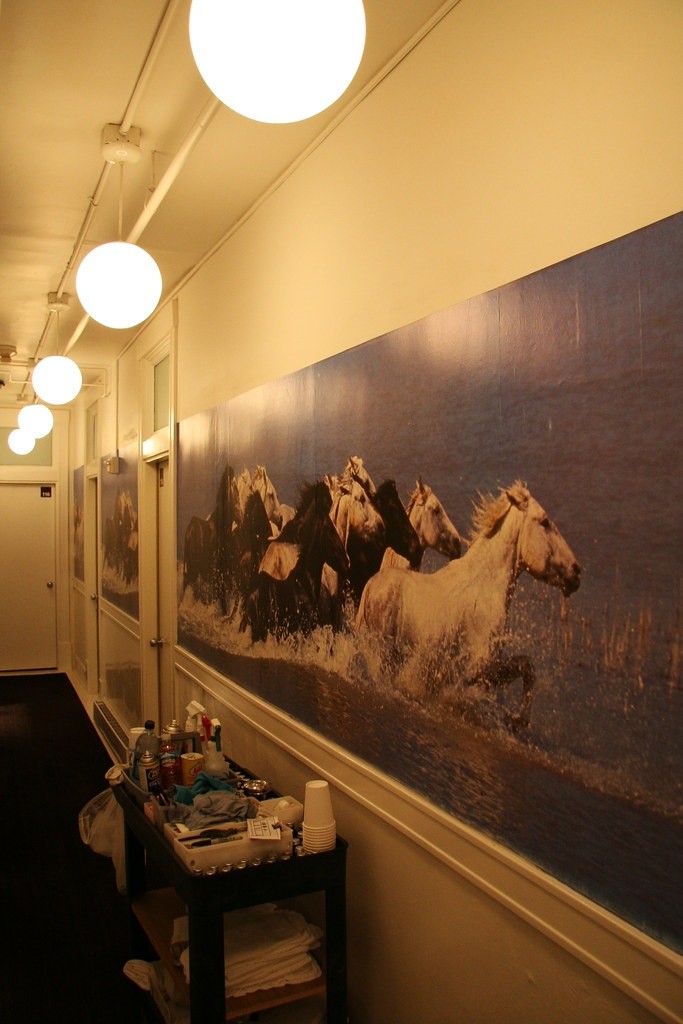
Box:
[183,455,586,741]
[103,489,139,591]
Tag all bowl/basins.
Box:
[244,780,272,800]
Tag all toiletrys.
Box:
[125,700,226,793]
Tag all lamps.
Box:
[8,310,83,456]
[76,163,163,330]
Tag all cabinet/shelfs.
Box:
[118,782,350,1024]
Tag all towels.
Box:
[180,909,322,999]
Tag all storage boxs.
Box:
[163,794,304,874]
[122,766,308,872]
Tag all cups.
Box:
[181,752,205,787]
[301,780,337,854]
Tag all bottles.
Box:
[159,733,180,788]
[138,750,160,793]
[132,720,159,785]
[161,720,183,736]
